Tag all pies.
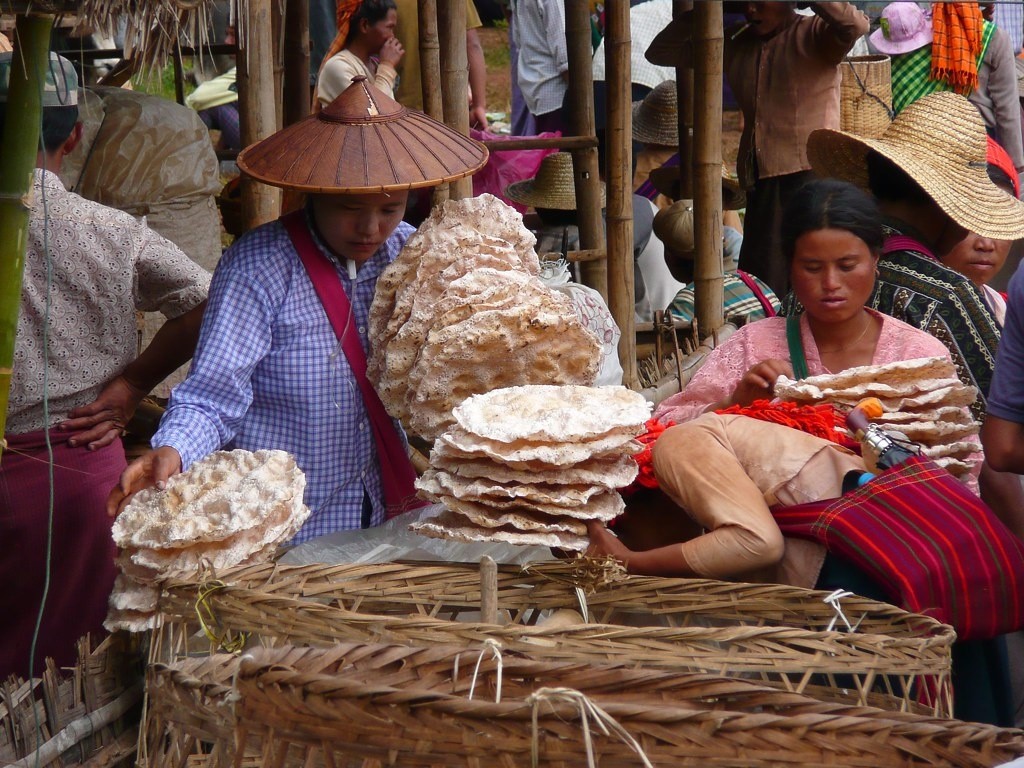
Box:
[367,193,601,445]
[408,384,653,553]
[774,355,981,479]
[101,447,311,633]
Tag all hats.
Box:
[870,1,933,55]
[503,154,606,211]
[237,74,490,195]
[986,133,1020,200]
[630,79,678,146]
[0,51,78,107]
[806,91,1024,240]
[651,199,728,253]
[649,159,746,211]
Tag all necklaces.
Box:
[819,316,873,353]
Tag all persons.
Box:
[632,79,743,239]
[651,199,783,328]
[550,411,1016,729]
[590,0,676,174]
[977,257,1024,476]
[506,153,606,284]
[805,91,1024,540]
[105,74,490,548]
[869,2,1024,174]
[653,177,984,496]
[185,64,240,150]
[0,49,214,689]
[510,0,570,136]
[313,0,405,109]
[640,0,870,300]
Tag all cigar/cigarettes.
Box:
[731,23,751,40]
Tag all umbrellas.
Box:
[848,411,916,469]
